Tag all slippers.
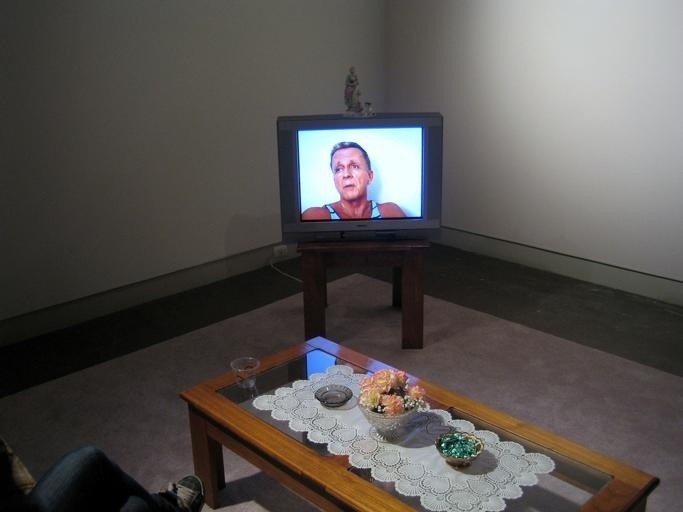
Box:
[165,474,205,511]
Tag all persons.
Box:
[302,142,407,220]
[0,446,206,512]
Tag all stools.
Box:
[291,238,430,350]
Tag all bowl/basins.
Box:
[434,431,484,467]
[314,384,353,407]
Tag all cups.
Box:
[230,356,260,389]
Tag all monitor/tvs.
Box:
[276,111,443,240]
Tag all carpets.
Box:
[3,269,683,512]
